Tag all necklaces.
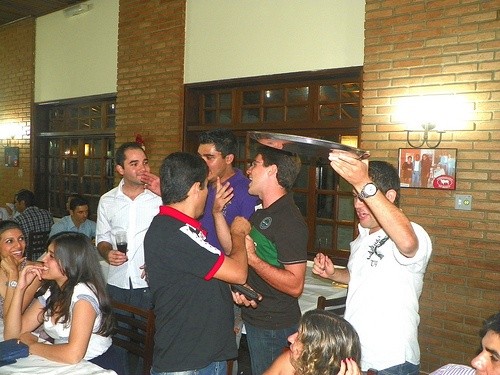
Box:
[0,258,27,278]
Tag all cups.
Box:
[116,232,128,256]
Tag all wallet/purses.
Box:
[0,338,30,367]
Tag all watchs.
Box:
[5,281,18,287]
[357,181,378,200]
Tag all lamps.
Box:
[0,118,25,148]
[395,96,465,150]
[63,3,93,18]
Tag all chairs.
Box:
[109,295,155,375]
[27,229,51,262]
[318,295,347,317]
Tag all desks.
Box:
[0,332,118,375]
[241,264,348,334]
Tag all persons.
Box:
[412,154,420,187]
[0,189,53,261]
[421,154,432,187]
[261,309,361,375]
[95,142,162,375]
[428,313,500,375]
[212,145,309,375]
[0,220,46,322]
[49,194,97,241]
[434,151,456,178]
[140,130,257,375]
[144,153,251,375]
[313,150,432,375]
[402,156,413,183]
[4,231,124,375]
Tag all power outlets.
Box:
[454,195,472,211]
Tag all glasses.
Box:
[247,160,263,168]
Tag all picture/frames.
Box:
[4,147,19,167]
[398,148,457,191]
